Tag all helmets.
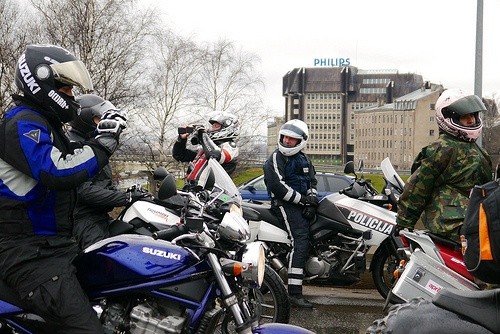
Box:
[9,43,94,125]
[73,94,116,138]
[208,112,240,141]
[435,86,487,142]
[278,119,309,156]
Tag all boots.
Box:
[285,268,313,309]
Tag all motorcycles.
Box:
[0,174,317,334]
[122,157,408,286]
[382,224,484,309]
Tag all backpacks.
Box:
[460,178,500,284]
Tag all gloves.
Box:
[393,222,414,237]
[126,187,150,205]
[302,196,317,221]
[297,195,318,206]
[190,124,206,145]
[89,108,128,153]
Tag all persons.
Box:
[263,119,319,309]
[64,94,155,252]
[392,87,495,244]
[172,112,242,193]
[0,42,129,334]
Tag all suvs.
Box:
[233,170,355,204]
